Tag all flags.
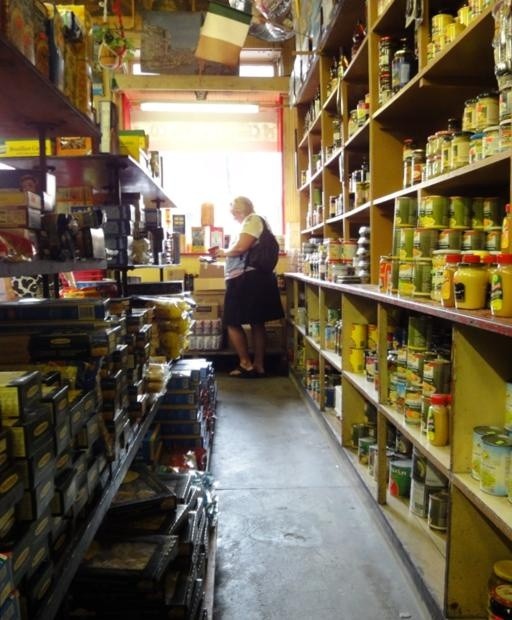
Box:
[195,0,253,68]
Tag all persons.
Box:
[210,197,286,379]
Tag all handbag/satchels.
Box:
[239,215,278,273]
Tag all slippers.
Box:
[228,365,256,377]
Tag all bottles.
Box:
[301,245,326,280]
[329,36,350,93]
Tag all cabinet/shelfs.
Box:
[0,1,217,620]
[282,0,512,614]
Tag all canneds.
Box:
[487,559,512,620]
